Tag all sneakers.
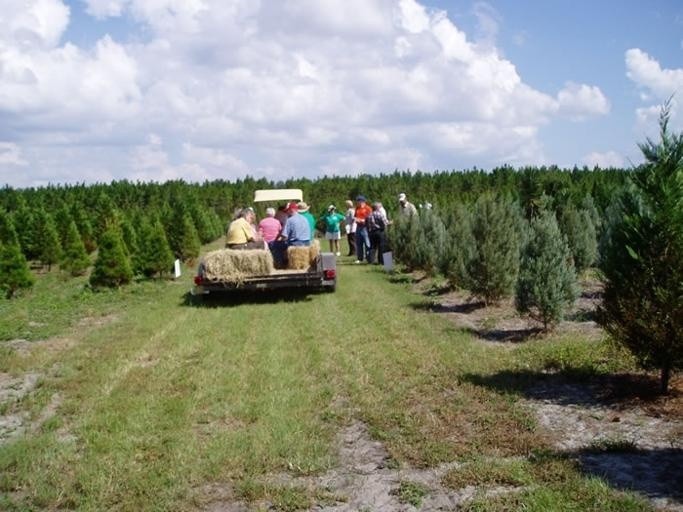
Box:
[336,252,363,264]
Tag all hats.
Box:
[297,202,310,213]
[357,196,365,201]
[284,202,299,212]
[399,193,405,201]
[328,205,335,212]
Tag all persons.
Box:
[225,192,420,269]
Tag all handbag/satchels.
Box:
[315,221,326,233]
[248,238,253,242]
[374,218,385,232]
